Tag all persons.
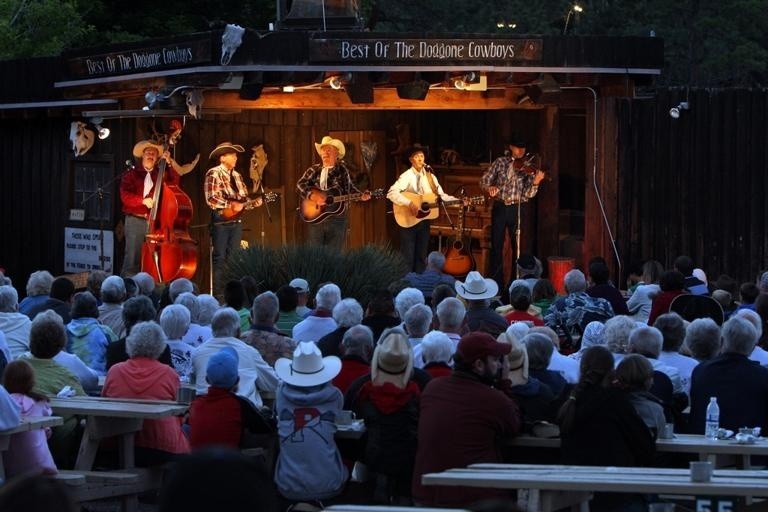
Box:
[0,249,768,512]
[478,130,546,290]
[118,139,180,276]
[203,141,263,297]
[385,145,474,275]
[297,135,372,253]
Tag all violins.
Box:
[513,153,553,182]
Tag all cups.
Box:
[176,386,196,405]
[336,409,357,425]
[690,461,713,482]
[659,422,674,438]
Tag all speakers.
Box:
[240,78,264,100]
[523,80,561,105]
[397,80,430,100]
[56,117,97,154]
[344,82,374,104]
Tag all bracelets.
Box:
[168,160,171,165]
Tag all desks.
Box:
[1,396,193,512]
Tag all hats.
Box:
[314,136,346,161]
[132,139,165,158]
[669,294,725,328]
[371,328,414,395]
[507,322,531,342]
[457,331,512,364]
[209,142,246,162]
[289,277,310,293]
[454,271,499,300]
[274,340,343,387]
[495,332,529,386]
[503,131,531,148]
[400,142,430,167]
[207,346,239,388]
[371,288,394,313]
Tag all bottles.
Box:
[704,396,720,440]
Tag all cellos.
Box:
[141,120,198,284]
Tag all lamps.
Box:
[94,124,111,140]
[330,72,352,90]
[669,101,689,120]
[454,71,475,91]
[144,90,165,105]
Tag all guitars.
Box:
[393,192,485,228]
[217,193,280,220]
[441,189,474,277]
[299,185,386,225]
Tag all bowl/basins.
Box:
[718,427,762,442]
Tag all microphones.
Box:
[422,162,433,173]
[126,160,135,169]
[338,159,351,176]
